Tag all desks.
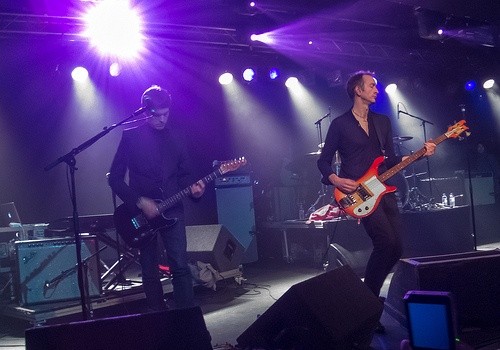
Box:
[257,204,471,272]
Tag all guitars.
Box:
[334,120,468,218]
[114,157,247,248]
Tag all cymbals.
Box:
[304,149,322,161]
[403,171,428,178]
[422,177,450,182]
[392,133,413,144]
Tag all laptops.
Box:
[0,202,50,227]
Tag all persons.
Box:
[108,85,204,311]
[316,71,436,301]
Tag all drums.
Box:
[386,170,410,208]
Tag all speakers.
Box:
[326,234,374,278]
[26,304,213,350]
[11,234,102,307]
[215,185,259,263]
[236,263,384,350]
[184,224,247,274]
[387,247,500,336]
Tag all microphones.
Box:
[45,280,50,287]
[329,106,331,119]
[397,104,399,119]
[144,95,155,114]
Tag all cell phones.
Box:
[402,290,458,350]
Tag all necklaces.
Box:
[352,109,368,121]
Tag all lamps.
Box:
[58,33,88,81]
[217,48,298,88]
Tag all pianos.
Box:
[47,214,172,294]
